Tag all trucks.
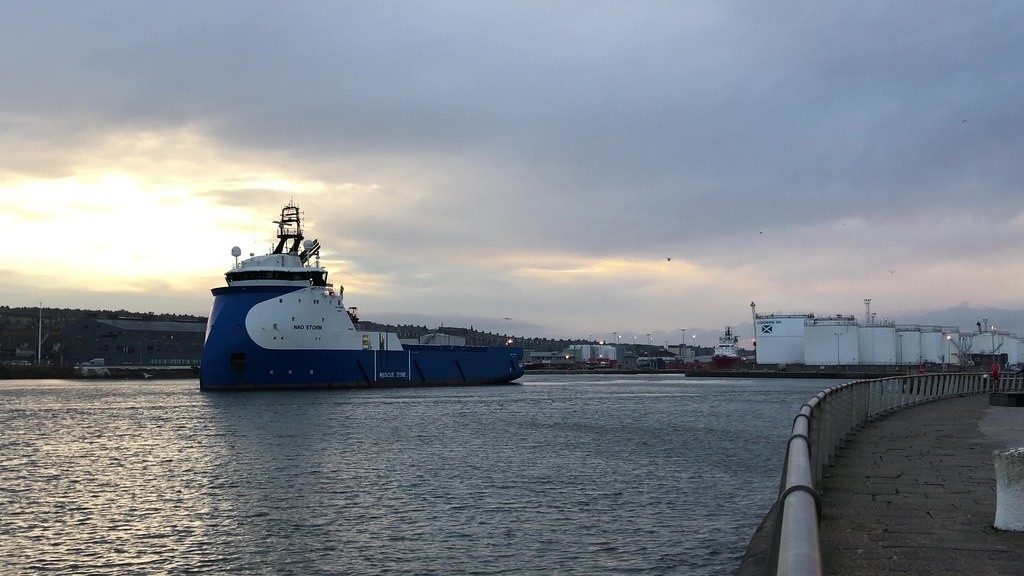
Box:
[89,359,104,367]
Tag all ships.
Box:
[695,325,747,370]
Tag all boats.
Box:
[199,197,524,391]
